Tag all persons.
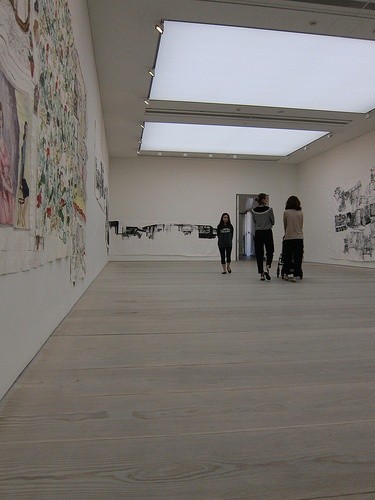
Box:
[282,196,304,281]
[252,193,275,280]
[216,213,233,274]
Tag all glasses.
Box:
[224,217,228,218]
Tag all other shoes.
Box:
[222,271,226,274]
[260,274,265,280]
[227,267,231,273]
[264,269,271,280]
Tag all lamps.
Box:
[148,65,155,77]
[182,153,188,157]
[328,133,334,139]
[208,154,213,158]
[155,21,165,34]
[232,155,237,159]
[365,113,373,120]
[136,148,141,155]
[143,98,151,106]
[138,137,143,144]
[140,122,145,129]
[304,146,310,151]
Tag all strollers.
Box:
[277,235,304,280]
